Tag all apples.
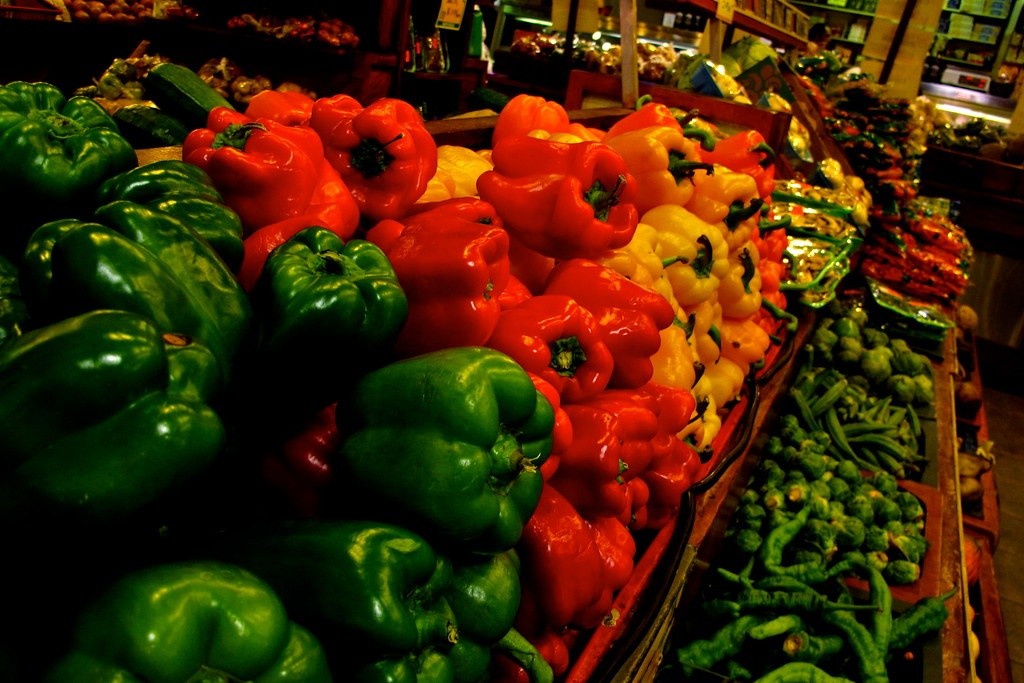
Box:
[62,0,198,22]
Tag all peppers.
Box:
[0,73,800,683]
[654,503,958,683]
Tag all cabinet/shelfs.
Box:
[0,1,1023,682]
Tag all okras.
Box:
[773,364,932,479]
